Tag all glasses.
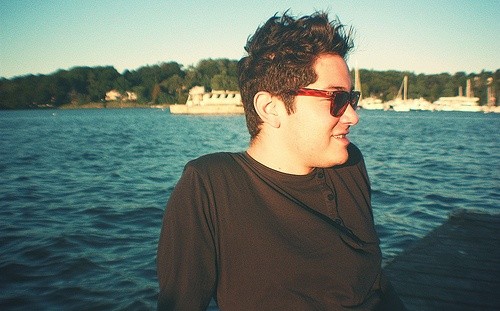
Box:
[289,87,360,118]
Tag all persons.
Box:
[157,8,409,311]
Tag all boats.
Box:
[353,66,384,111]
[385,76,433,110]
[169,84,244,115]
[428,79,500,112]
